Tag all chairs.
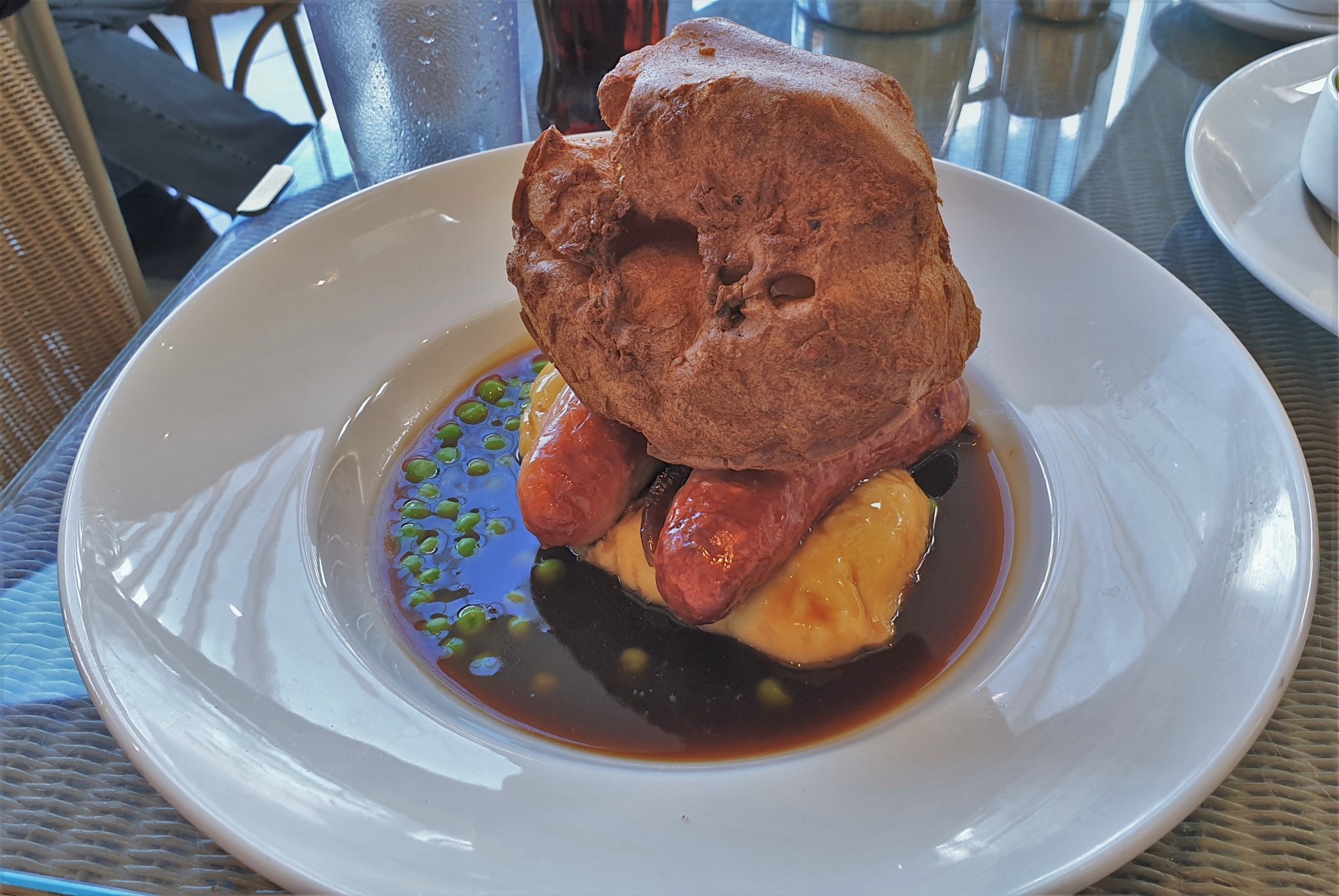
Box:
[137,0,325,221]
[0,0,151,489]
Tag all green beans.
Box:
[401,359,797,709]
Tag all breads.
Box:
[508,17,980,469]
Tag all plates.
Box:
[54,130,1319,895]
[1184,33,1338,336]
[1195,1,1339,43]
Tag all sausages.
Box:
[655,378,971,623]
[519,380,664,546]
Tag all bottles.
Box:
[529,0,668,137]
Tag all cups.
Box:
[1019,0,1109,23]
[796,0,979,32]
[1301,65,1339,224]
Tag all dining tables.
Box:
[0,0,1339,896]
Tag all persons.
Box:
[48,0,315,244]
